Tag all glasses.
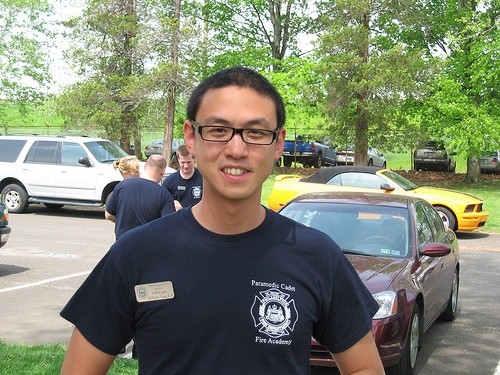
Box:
[190,119,279,145]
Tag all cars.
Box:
[101,138,136,159]
[144,137,185,162]
[267,165,490,235]
[480,150,500,174]
[334,142,387,170]
[276,191,461,375]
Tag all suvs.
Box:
[0,134,177,213]
[413,139,456,172]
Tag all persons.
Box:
[59,66,386,375]
[105,155,175,242]
[162,145,203,211]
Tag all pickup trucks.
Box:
[281,134,338,169]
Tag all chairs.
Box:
[309,207,407,255]
